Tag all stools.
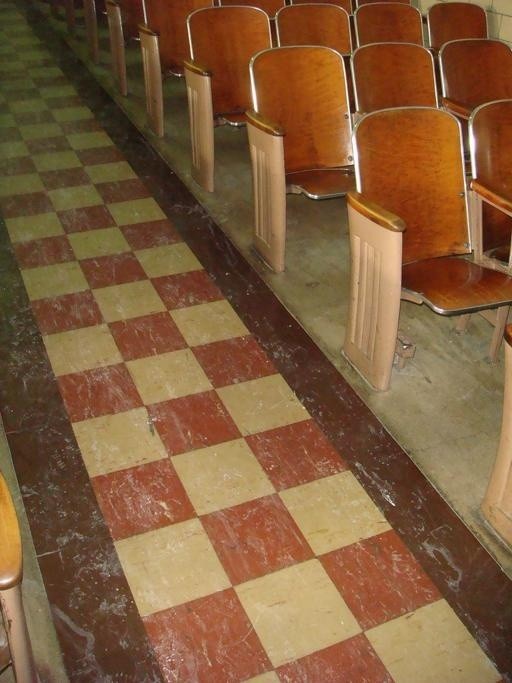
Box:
[2,477,40,682]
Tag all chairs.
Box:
[468,98,511,272]
[80,0,490,196]
[349,42,472,178]
[437,39,511,121]
[248,45,357,274]
[341,106,512,392]
